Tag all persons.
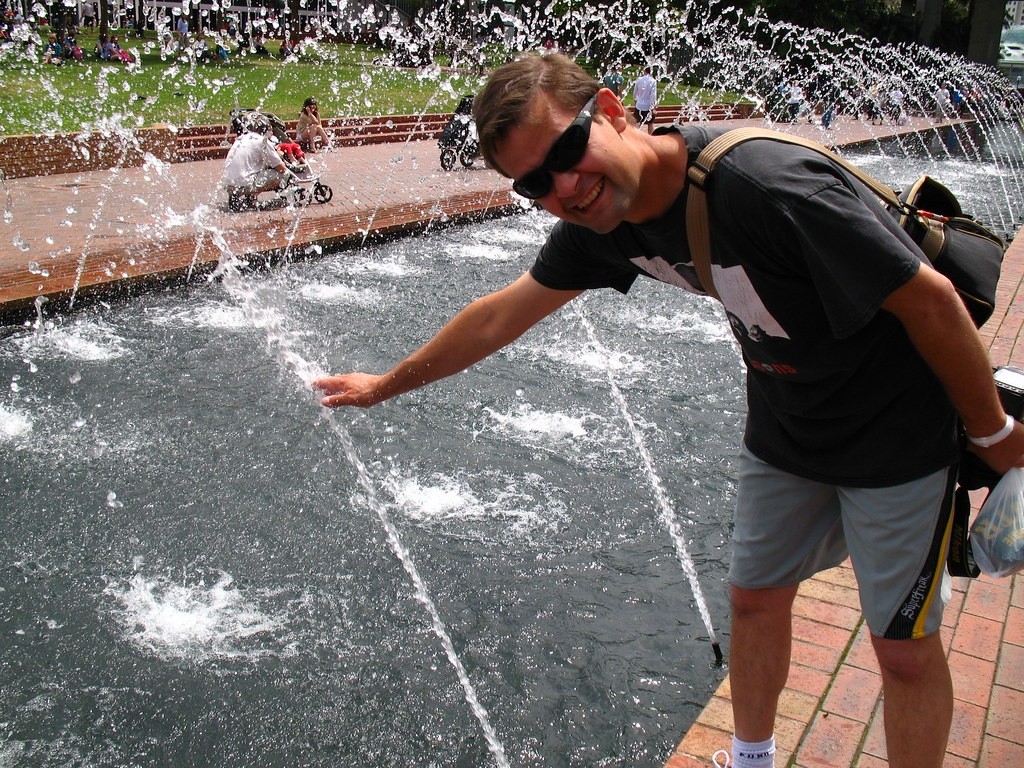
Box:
[312,56,1023,767]
[86,0,1023,211]
[0,0,83,67]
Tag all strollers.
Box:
[439,94,480,170]
[229,108,333,206]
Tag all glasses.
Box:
[513,92,597,200]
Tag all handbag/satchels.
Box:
[885,176,1005,330]
[968,466,1024,578]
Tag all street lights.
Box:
[1015,76,1021,91]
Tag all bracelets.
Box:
[964,415,1014,448]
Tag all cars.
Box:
[1001,43,1024,61]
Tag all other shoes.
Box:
[242,198,257,210]
[229,194,239,212]
